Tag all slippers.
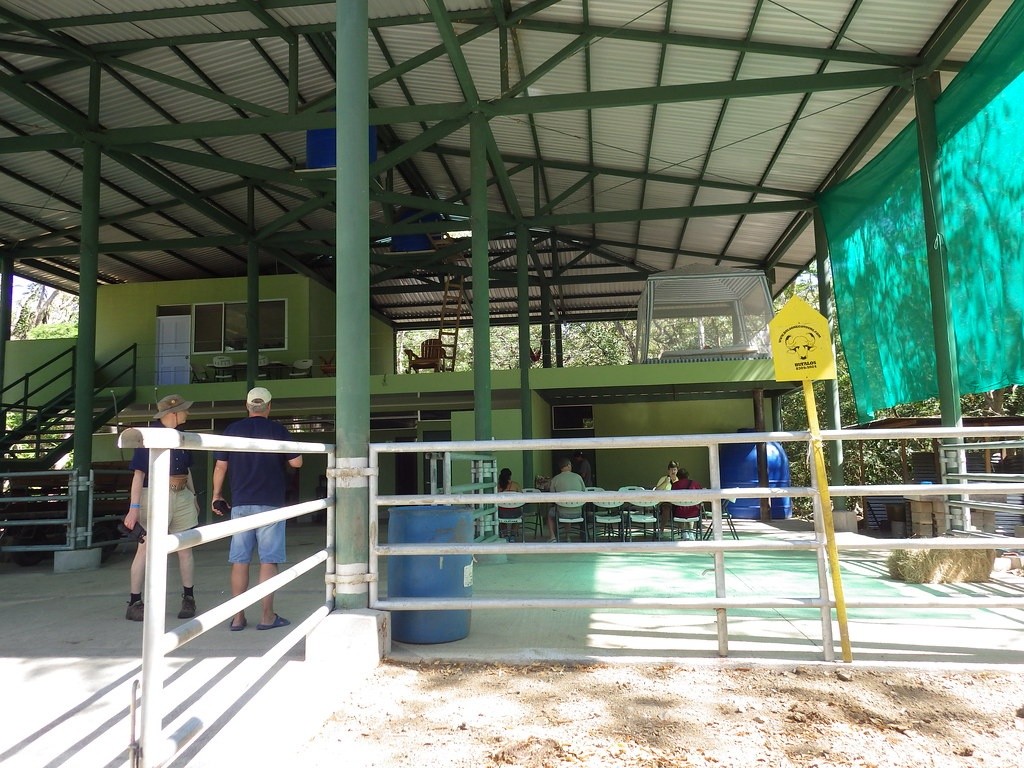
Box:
[256,613,291,629]
[230,617,247,630]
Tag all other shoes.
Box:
[566,535,571,541]
[656,531,664,539]
[682,532,689,540]
[548,538,557,543]
[688,533,695,541]
[506,535,515,542]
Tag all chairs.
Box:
[593,490,626,543]
[618,486,647,539]
[670,502,704,541]
[496,491,525,544]
[585,487,615,537]
[625,490,660,542]
[704,487,740,540]
[404,338,446,373]
[653,487,683,539]
[213,355,237,382]
[520,488,543,540]
[554,490,589,543]
[289,359,313,378]
[190,364,212,383]
[258,355,271,380]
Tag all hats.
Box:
[153,395,194,418]
[247,387,272,406]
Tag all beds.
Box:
[660,345,759,358]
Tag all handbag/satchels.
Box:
[171,475,189,490]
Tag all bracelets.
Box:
[130,504,140,509]
[193,493,197,497]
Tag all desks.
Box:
[206,361,284,380]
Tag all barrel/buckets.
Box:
[387,507,476,644]
[719,427,793,520]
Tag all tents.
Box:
[625,261,778,366]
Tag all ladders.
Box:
[434,273,464,372]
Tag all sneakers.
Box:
[178,593,196,617]
[125,600,144,621]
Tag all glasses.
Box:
[567,464,573,467]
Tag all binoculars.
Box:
[117,513,146,544]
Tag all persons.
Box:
[574,453,592,489]
[209,387,304,631]
[122,394,201,622]
[670,467,704,540]
[546,455,586,543]
[497,467,525,543]
[653,460,690,540]
